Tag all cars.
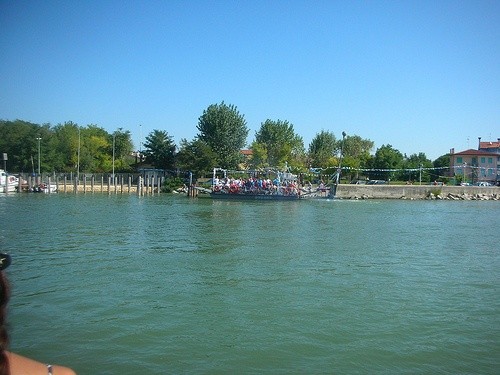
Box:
[476,182,489,187]
[349,180,385,184]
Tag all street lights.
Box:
[35,137,42,185]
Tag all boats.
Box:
[0,169,28,193]
[194,186,301,200]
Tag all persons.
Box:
[0,251,77,375]
[433,180,438,186]
[214,176,323,197]
[442,182,446,186]
[366,176,369,181]
[176,184,188,193]
[38,180,46,191]
